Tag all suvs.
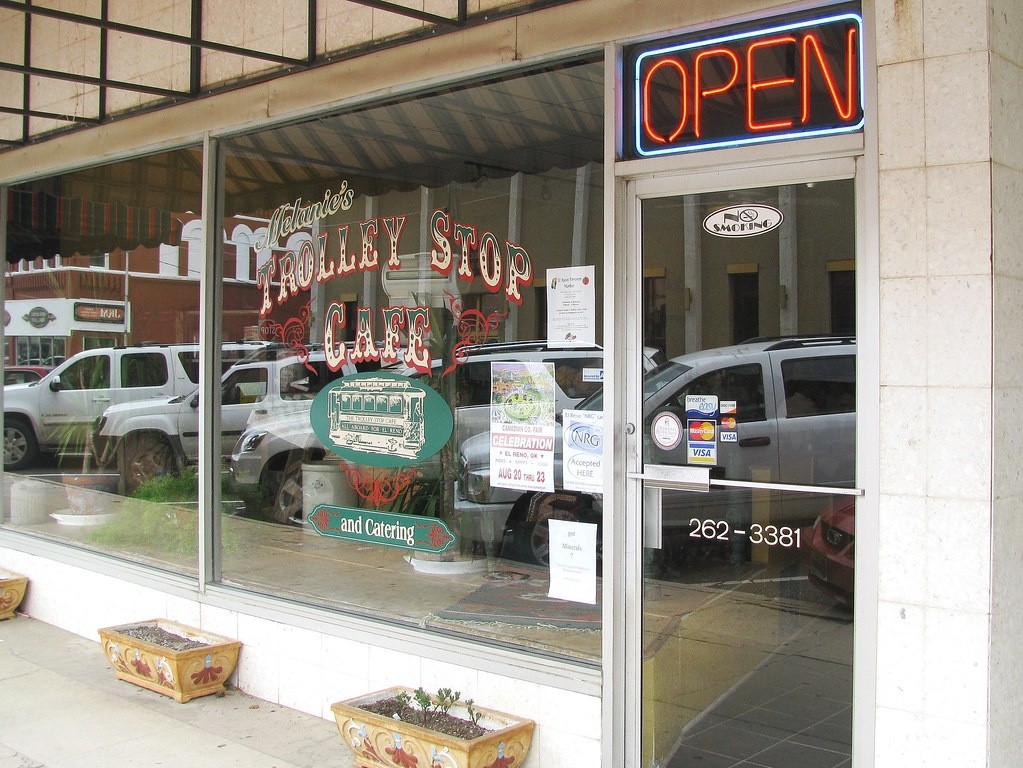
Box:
[4,340,276,471]
[92,339,430,495]
[452,334,857,568]
[229,340,687,528]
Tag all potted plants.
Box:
[83,450,270,566]
[358,292,496,575]
[330,685,535,768]
[34,259,151,515]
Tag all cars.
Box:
[800,500,855,609]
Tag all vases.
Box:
[0,566,29,622]
[97,618,242,704]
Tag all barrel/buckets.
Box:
[300,460,358,534]
